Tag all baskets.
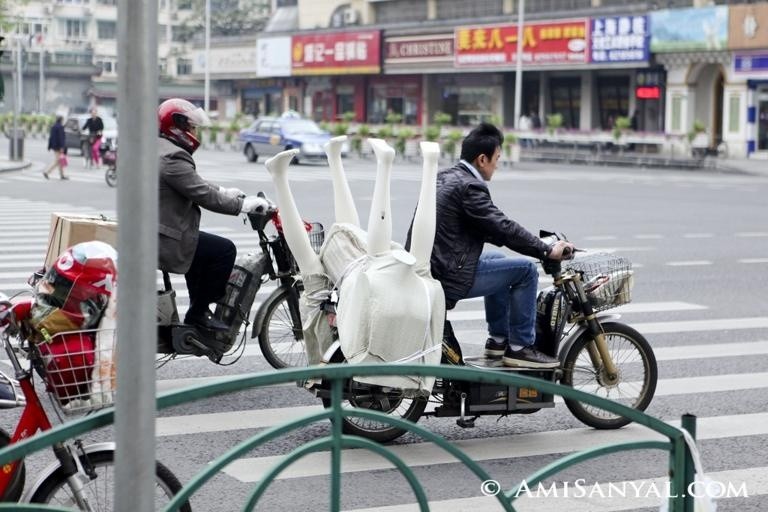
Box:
[569,253,633,313]
[303,222,325,253]
[33,328,116,413]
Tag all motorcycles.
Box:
[0,287,194,512]
[300,229,658,443]
[26,191,339,384]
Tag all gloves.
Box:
[239,196,268,215]
[217,185,245,199]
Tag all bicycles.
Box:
[99,138,117,187]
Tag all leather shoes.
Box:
[183,308,229,331]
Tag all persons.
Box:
[520,113,535,148]
[337,138,446,392]
[404,123,573,369]
[628,110,639,151]
[530,109,542,130]
[43,115,68,179]
[264,134,363,369]
[83,108,103,166]
[157,97,268,331]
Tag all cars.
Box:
[235,107,347,165]
[63,115,119,155]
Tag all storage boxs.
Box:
[43,213,119,274]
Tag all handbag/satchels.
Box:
[584,269,633,308]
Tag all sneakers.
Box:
[484,337,505,355]
[502,344,561,368]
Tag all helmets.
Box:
[159,98,211,155]
